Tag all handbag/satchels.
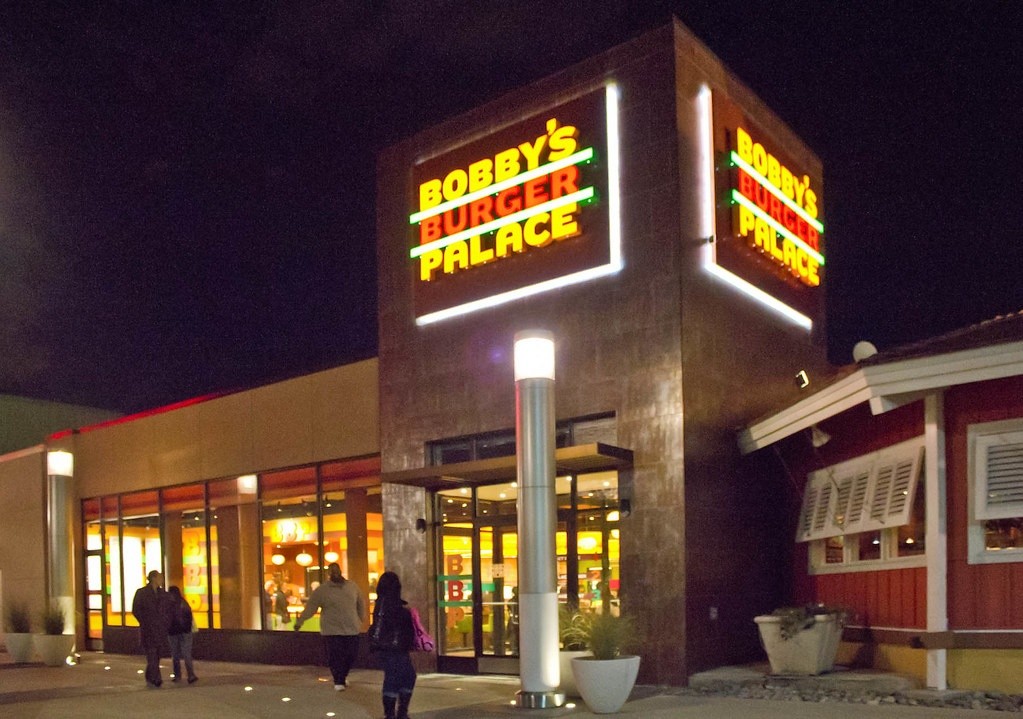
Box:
[408,607,434,651]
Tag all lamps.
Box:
[619,498,630,518]
[810,424,833,448]
[415,518,426,533]
[795,370,809,392]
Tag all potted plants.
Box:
[568,601,642,714]
[32,601,74,666]
[753,605,849,674]
[558,593,619,695]
[1,592,35,661]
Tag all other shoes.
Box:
[345,678,350,686]
[189,677,198,683]
[146,679,162,688]
[334,685,345,691]
[172,678,181,682]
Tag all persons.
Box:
[293,563,366,691]
[133,570,176,687]
[367,572,417,719]
[167,586,199,684]
